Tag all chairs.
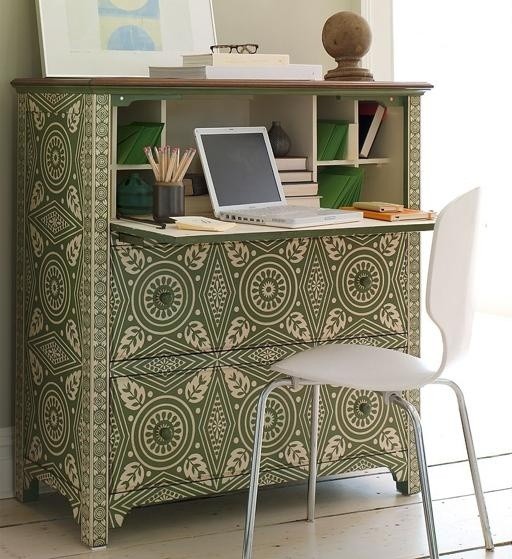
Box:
[240,187,495,554]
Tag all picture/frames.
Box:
[33,2,221,81]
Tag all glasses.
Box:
[210,44,258,54]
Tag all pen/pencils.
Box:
[142,146,196,184]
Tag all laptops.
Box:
[194,125,365,230]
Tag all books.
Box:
[285,197,324,209]
[357,99,388,160]
[274,153,323,170]
[145,65,324,82]
[280,183,320,196]
[353,200,405,213]
[339,207,438,222]
[276,171,314,183]
[181,53,290,66]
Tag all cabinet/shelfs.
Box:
[10,76,435,553]
